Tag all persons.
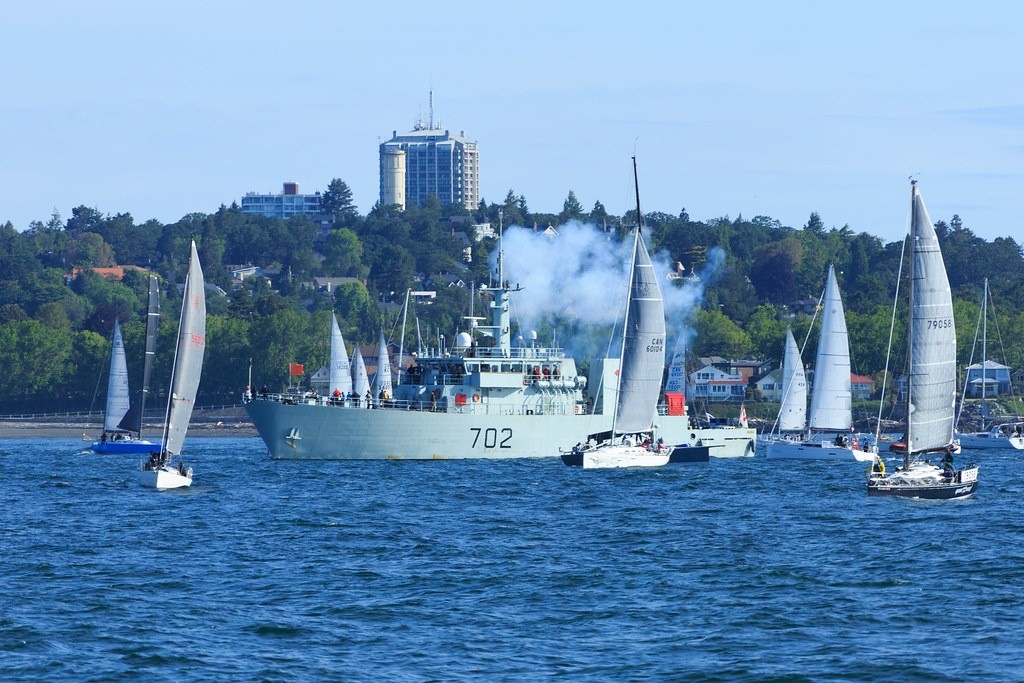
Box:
[245,364,462,412]
[527,364,560,386]
[577,433,699,454]
[784,431,805,441]
[864,456,885,478]
[101,431,132,443]
[835,433,869,452]
[148,451,185,474]
[940,444,955,483]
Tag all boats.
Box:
[242,208,758,462]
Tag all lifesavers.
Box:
[472,393,480,402]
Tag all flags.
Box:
[739,404,748,428]
[290,364,304,376]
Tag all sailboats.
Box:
[764,176,1024,500]
[91,272,165,456]
[559,226,677,470]
[137,229,207,487]
